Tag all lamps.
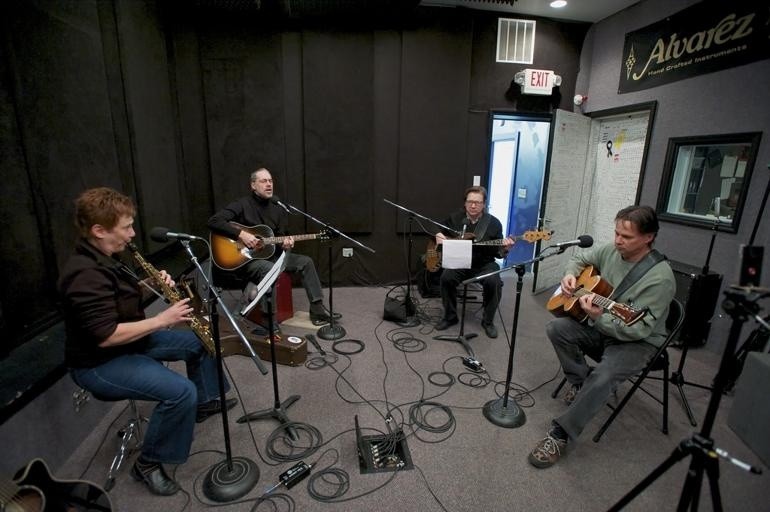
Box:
[551,298,686,443]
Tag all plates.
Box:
[683,147,710,214]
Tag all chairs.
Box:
[528,430,569,468]
[564,383,583,407]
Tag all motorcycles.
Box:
[434,238,506,363]
[237,248,301,442]
[630,344,718,427]
[603,287,768,511]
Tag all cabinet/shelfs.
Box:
[103,419,135,479]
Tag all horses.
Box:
[738,244,766,288]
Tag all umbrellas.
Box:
[309,308,343,321]
[435,315,459,331]
[481,318,497,338]
[195,398,238,423]
[127,459,181,496]
[261,320,283,334]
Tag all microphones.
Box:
[93,394,150,492]
[247,272,293,329]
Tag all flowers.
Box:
[269,193,290,216]
[548,235,595,253]
[460,217,472,240]
[150,225,200,246]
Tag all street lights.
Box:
[383,297,407,322]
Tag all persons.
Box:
[57,187,239,497]
[207,169,343,323]
[434,187,516,338]
[529,206,677,469]
